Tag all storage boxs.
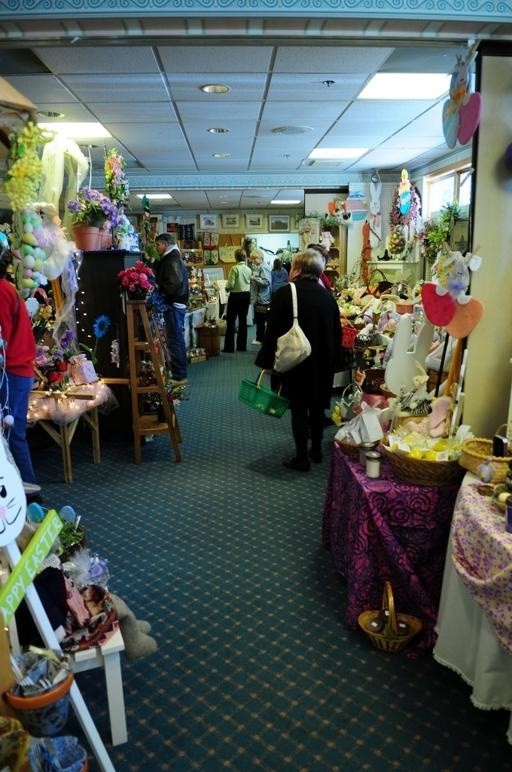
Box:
[395,304,412,315]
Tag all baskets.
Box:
[382,441,457,488]
[237,369,287,420]
[357,581,421,652]
[342,326,360,349]
[461,437,511,481]
[335,438,357,454]
[350,315,375,345]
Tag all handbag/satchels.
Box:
[254,303,269,314]
[274,320,311,372]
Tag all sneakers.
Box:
[282,457,310,472]
[309,450,322,463]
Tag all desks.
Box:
[25,380,122,482]
[431,470,510,745]
[320,432,464,662]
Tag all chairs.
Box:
[0,565,129,747]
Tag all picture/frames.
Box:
[221,213,239,228]
[269,214,291,232]
[200,214,219,230]
[245,213,264,228]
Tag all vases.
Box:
[6,645,75,739]
[126,286,147,301]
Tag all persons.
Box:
[220,249,252,353]
[268,250,292,289]
[0,256,44,506]
[255,249,341,471]
[248,250,271,346]
[155,234,188,388]
[307,245,338,438]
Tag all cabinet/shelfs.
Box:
[195,319,220,358]
[74,248,147,446]
[179,241,203,297]
[183,306,207,350]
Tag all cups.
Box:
[118,240,130,250]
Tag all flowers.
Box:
[67,187,124,251]
[118,260,157,291]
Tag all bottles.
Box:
[190,271,196,282]
[364,451,382,478]
[196,269,202,281]
[359,442,376,466]
